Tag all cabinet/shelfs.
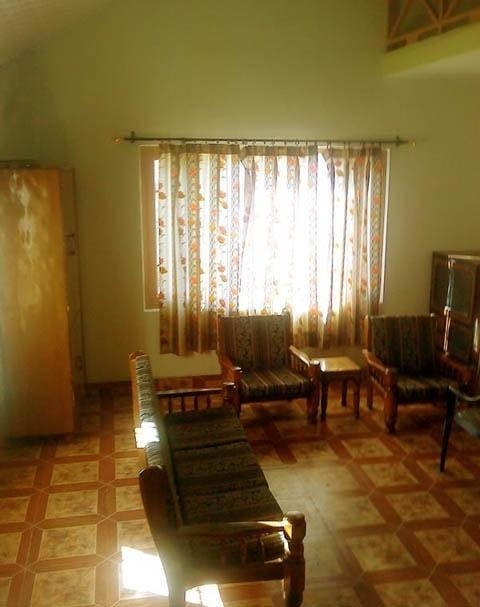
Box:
[429,252,480,391]
[1,163,83,441]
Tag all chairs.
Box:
[363,311,469,436]
[439,383,479,474]
[213,304,325,427]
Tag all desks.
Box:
[312,356,363,424]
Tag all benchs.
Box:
[128,351,308,607]
